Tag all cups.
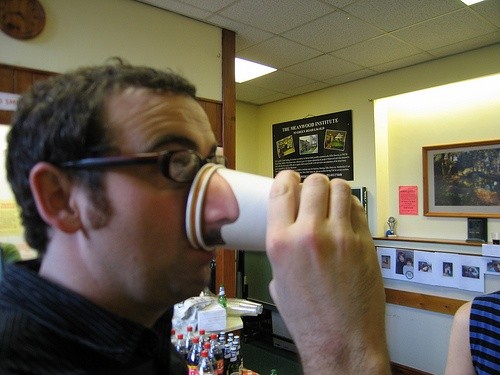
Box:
[184,162,302,254]
[491,232,500,245]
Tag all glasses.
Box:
[48,149,218,184]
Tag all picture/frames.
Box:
[422,139,500,218]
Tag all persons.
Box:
[487,262,500,272]
[397,252,413,274]
[0,65,392,375]
[445,264,451,274]
[445,289,500,375]
[419,264,432,272]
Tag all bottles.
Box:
[170,327,244,375]
[270,369,278,375]
[216,283,227,309]
